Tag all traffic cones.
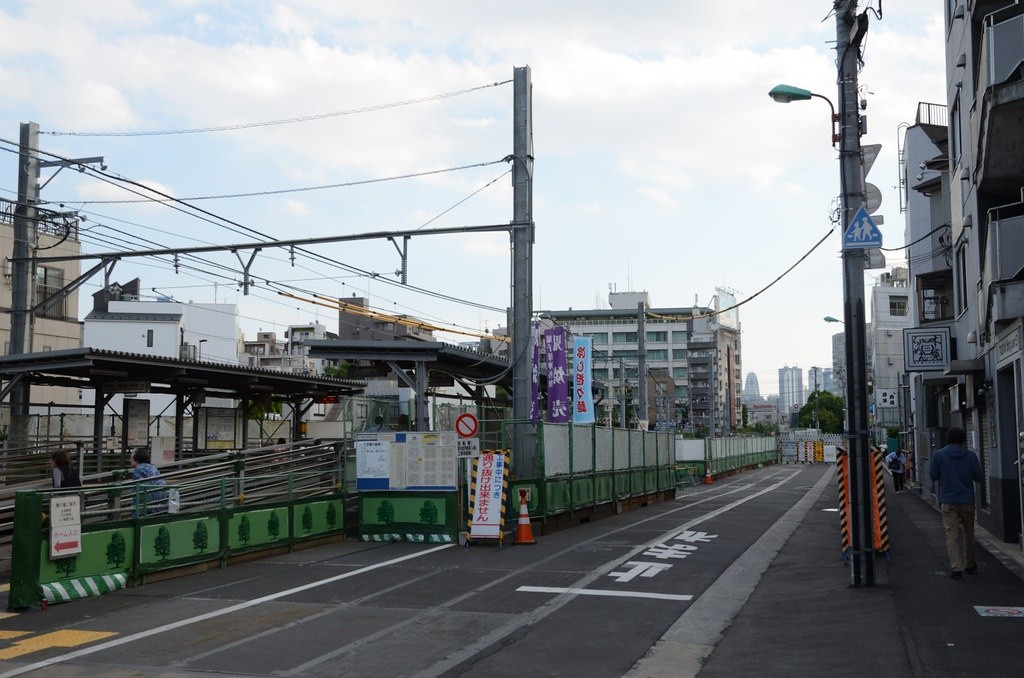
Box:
[511,498,538,545]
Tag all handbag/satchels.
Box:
[889,458,899,469]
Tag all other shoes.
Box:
[895,491,900,494]
[965,562,977,573]
[950,572,963,580]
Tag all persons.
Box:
[366,416,391,433]
[393,413,409,431]
[49,449,89,501]
[129,447,169,519]
[272,437,289,468]
[878,440,888,458]
[886,447,906,494]
[928,426,984,579]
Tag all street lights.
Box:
[769,84,876,587]
